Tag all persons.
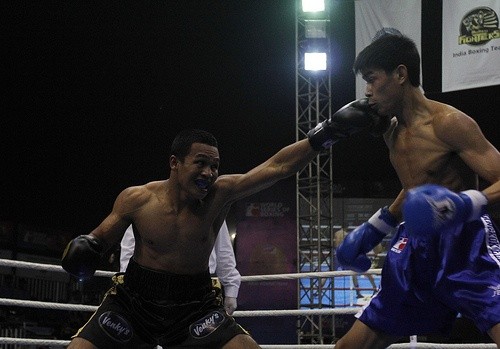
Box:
[115,214,241,317]
[61,96,375,349]
[333,28,500,349]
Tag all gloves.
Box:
[337,203,399,273]
[401,184,489,238]
[60,235,105,281]
[308,97,390,154]
